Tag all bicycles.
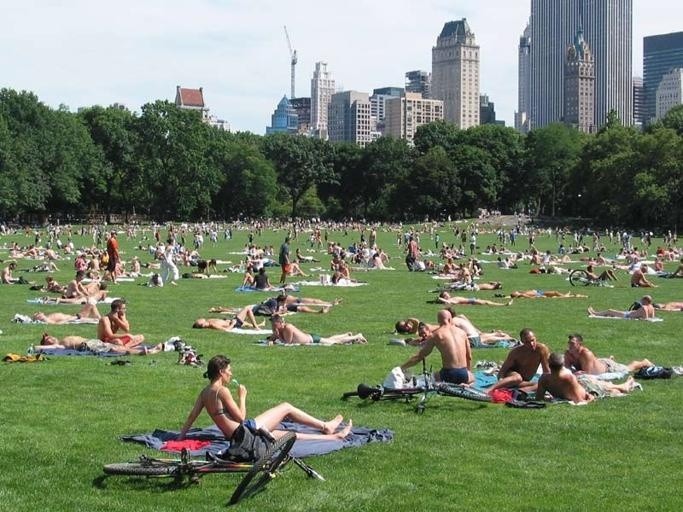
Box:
[570,268,615,289]
[337,356,494,417]
[98,424,329,505]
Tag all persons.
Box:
[176,355,353,441]
[1,207,683,404]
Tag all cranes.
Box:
[281,24,299,100]
